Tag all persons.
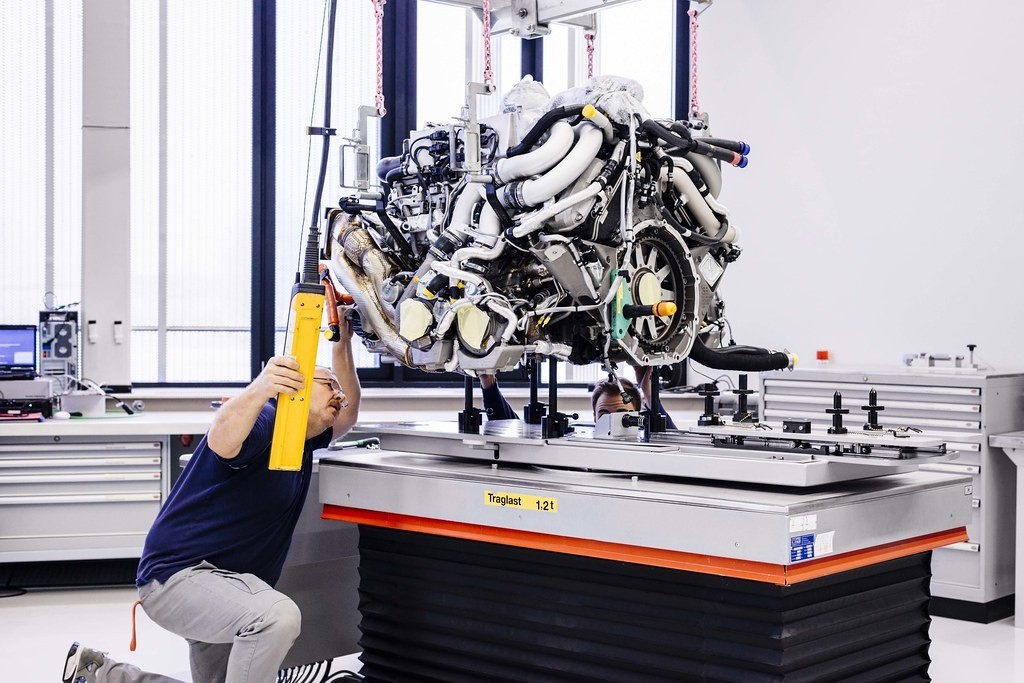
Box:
[592,364,677,429]
[62,305,362,683]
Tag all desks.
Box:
[319,451,972,683]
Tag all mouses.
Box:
[54,411,71,421]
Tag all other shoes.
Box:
[61,641,104,683]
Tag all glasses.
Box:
[313,377,345,394]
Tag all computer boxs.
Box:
[38,311,78,394]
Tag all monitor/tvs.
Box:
[0,325,36,369]
